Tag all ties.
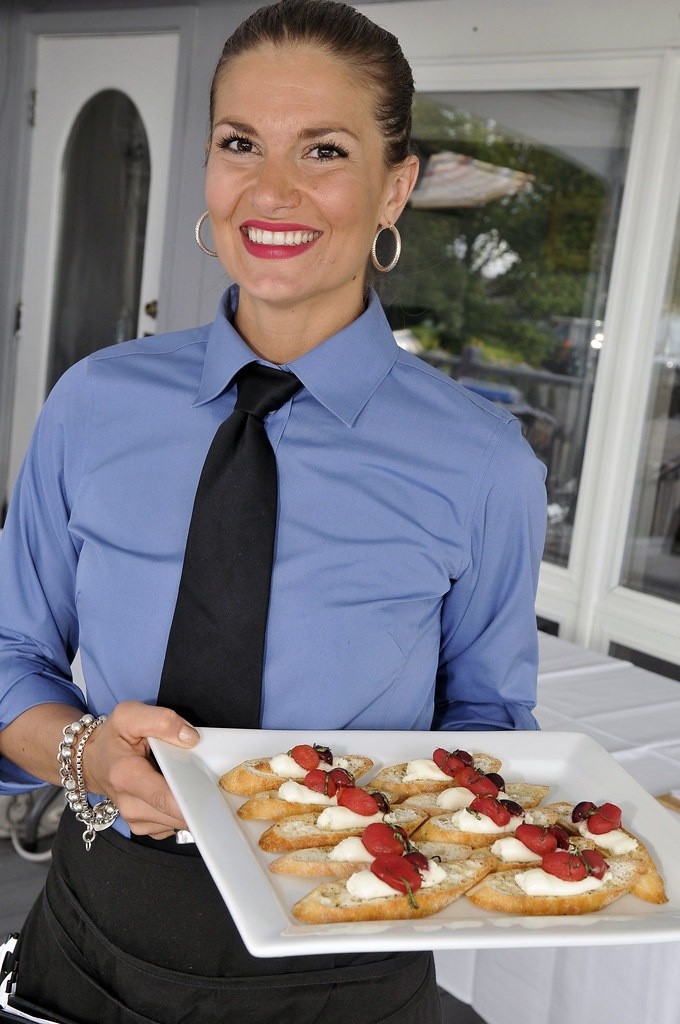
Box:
[129,358,306,857]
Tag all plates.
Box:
[147,727,680,960]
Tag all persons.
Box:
[0,1,548,1024]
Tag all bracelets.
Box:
[56,712,121,851]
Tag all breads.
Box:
[219,743,669,926]
